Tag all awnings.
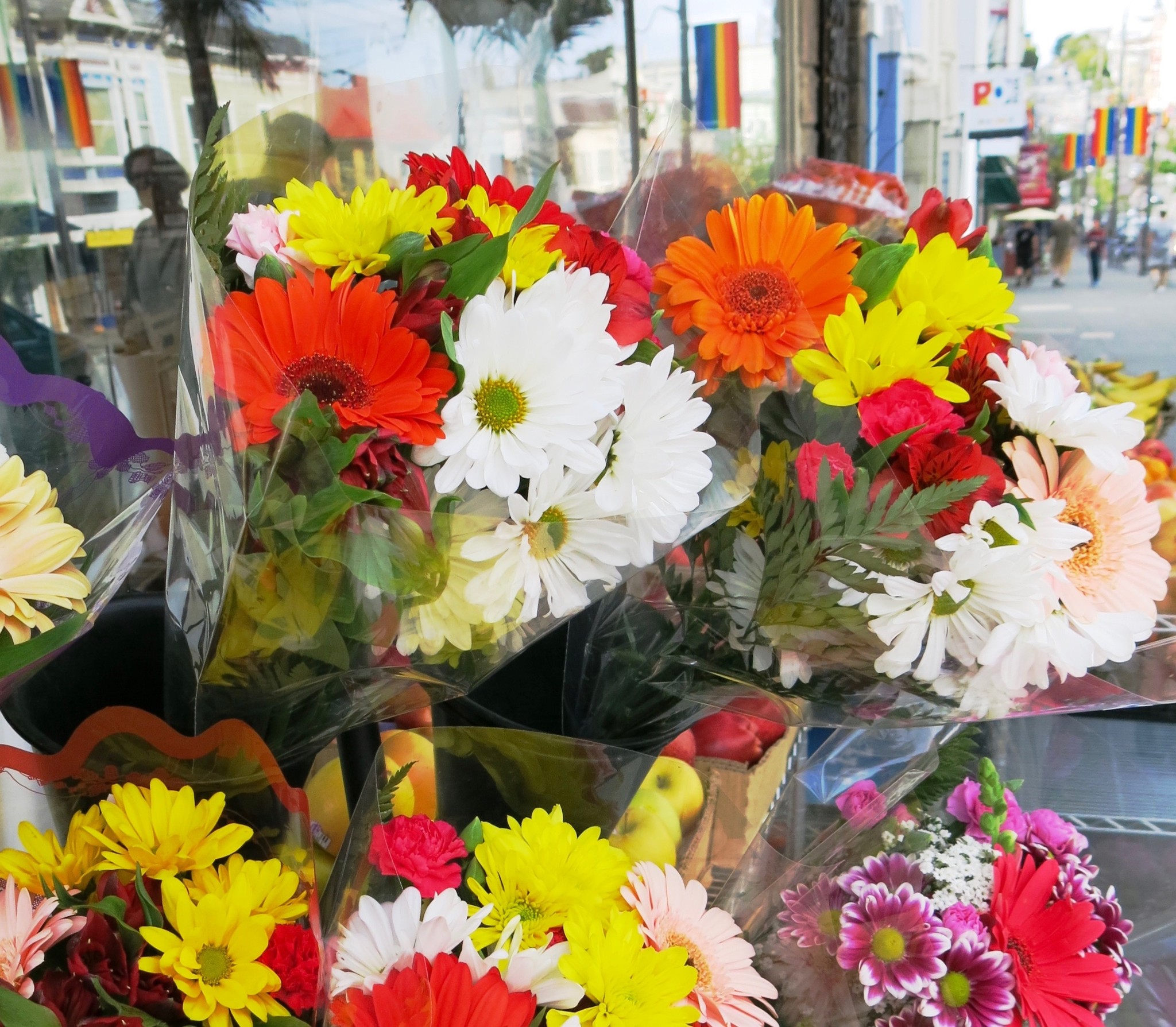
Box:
[982,155,1017,204]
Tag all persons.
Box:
[1046,215,1079,288]
[1013,221,1041,288]
[1144,210,1174,294]
[1081,218,1107,287]
[108,148,209,432]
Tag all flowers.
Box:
[0,103,1175,1027]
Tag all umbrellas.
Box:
[1005,206,1060,221]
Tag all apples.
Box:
[284,682,436,915]
[610,690,791,882]
